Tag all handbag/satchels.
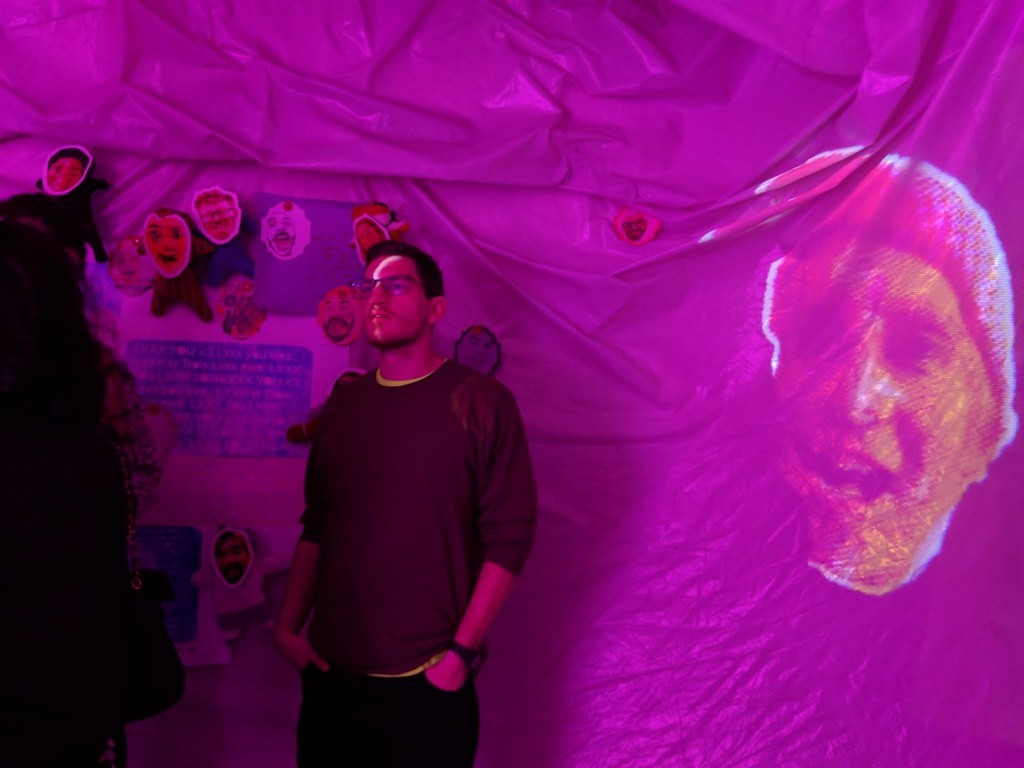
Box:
[115,565,185,723]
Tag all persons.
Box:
[46,148,88,191]
[214,533,249,584]
[275,239,538,768]
[0,193,165,768]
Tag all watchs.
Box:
[448,640,482,675]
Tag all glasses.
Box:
[348,273,427,301]
